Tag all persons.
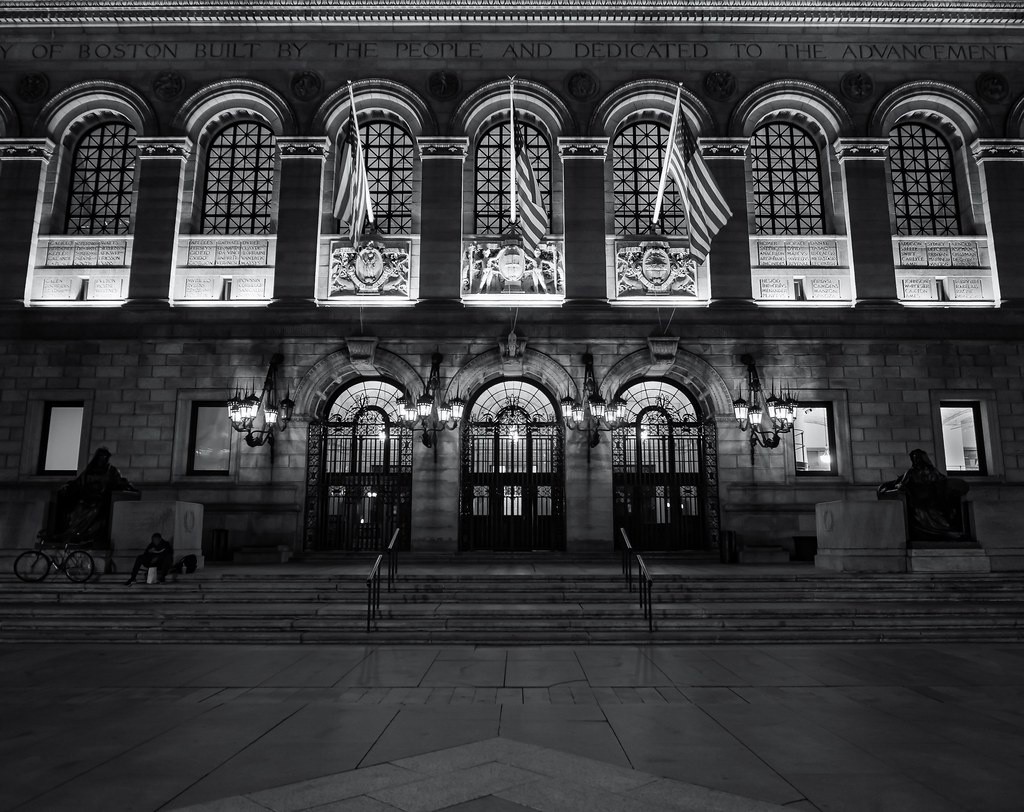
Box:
[67,448,138,547]
[123,533,173,587]
[878,449,960,542]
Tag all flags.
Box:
[666,101,733,266]
[513,100,548,257]
[332,102,367,249]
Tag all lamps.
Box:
[226,349,296,449]
[731,353,798,466]
[559,355,626,466]
[395,345,465,449]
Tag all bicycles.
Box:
[13,528,95,583]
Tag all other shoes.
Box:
[125,577,136,586]
[160,578,165,584]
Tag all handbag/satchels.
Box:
[147,567,158,584]
[170,554,197,575]
